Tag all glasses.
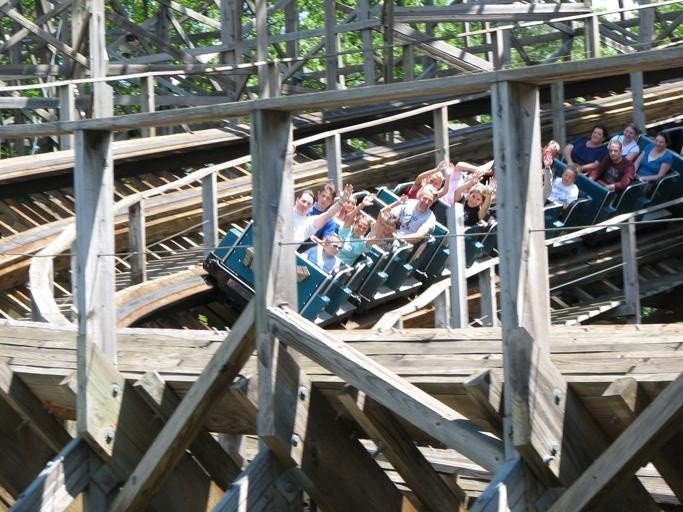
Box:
[334,245,342,249]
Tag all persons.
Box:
[390,159,497,244]
[633,132,673,193]
[542,141,579,212]
[564,125,609,173]
[292,183,408,274]
[607,124,639,161]
[589,139,635,191]
